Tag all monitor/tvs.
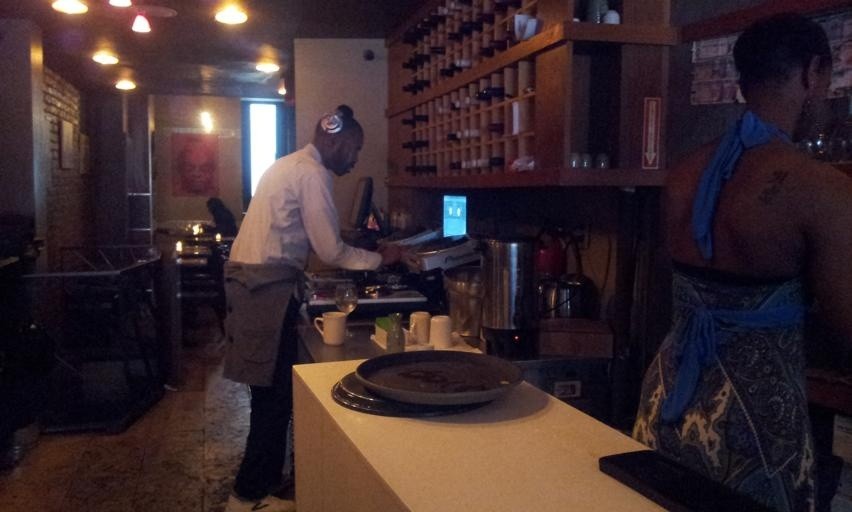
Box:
[440,194,468,239]
[347,177,384,231]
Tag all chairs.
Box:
[176,246,228,340]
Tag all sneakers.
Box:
[224,495,296,512]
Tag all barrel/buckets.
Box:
[558,284,582,318]
[534,279,558,317]
[441,266,489,338]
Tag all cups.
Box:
[408,311,454,349]
[568,151,609,169]
[334,284,358,316]
[313,312,347,347]
[604,10,620,24]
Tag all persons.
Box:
[632,13,852,511]
[223,105,402,511]
[199,197,237,237]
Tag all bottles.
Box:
[401,13,519,173]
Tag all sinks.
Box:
[48,241,162,277]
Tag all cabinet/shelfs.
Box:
[382,0,679,225]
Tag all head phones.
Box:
[322,108,348,136]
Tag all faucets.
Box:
[20,234,47,273]
